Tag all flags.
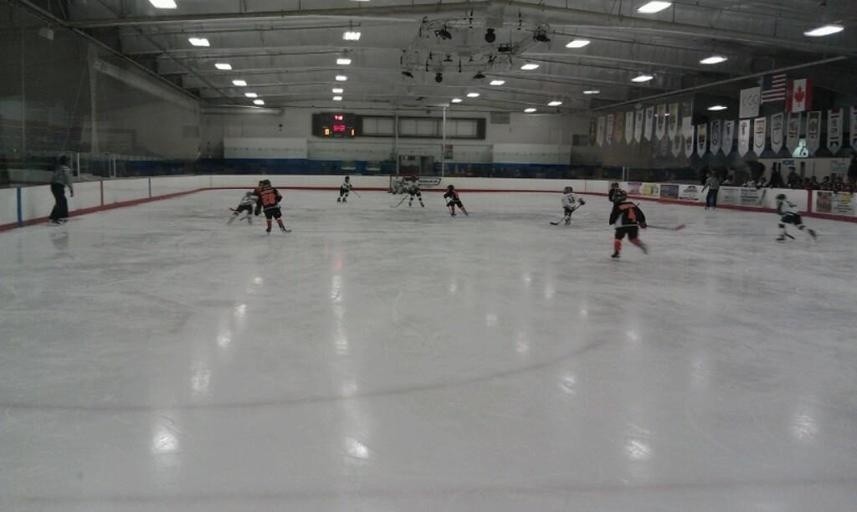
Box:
[760,74,812,112]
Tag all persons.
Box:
[227,180,288,232]
[444,185,468,216]
[561,187,584,225]
[776,194,817,240]
[701,166,857,209]
[337,177,353,203]
[407,176,425,208]
[49,156,73,225]
[608,182,648,258]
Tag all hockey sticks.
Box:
[390,193,409,207]
[615,223,686,231]
[785,231,794,240]
[549,203,582,225]
[445,197,456,216]
[234,211,264,222]
